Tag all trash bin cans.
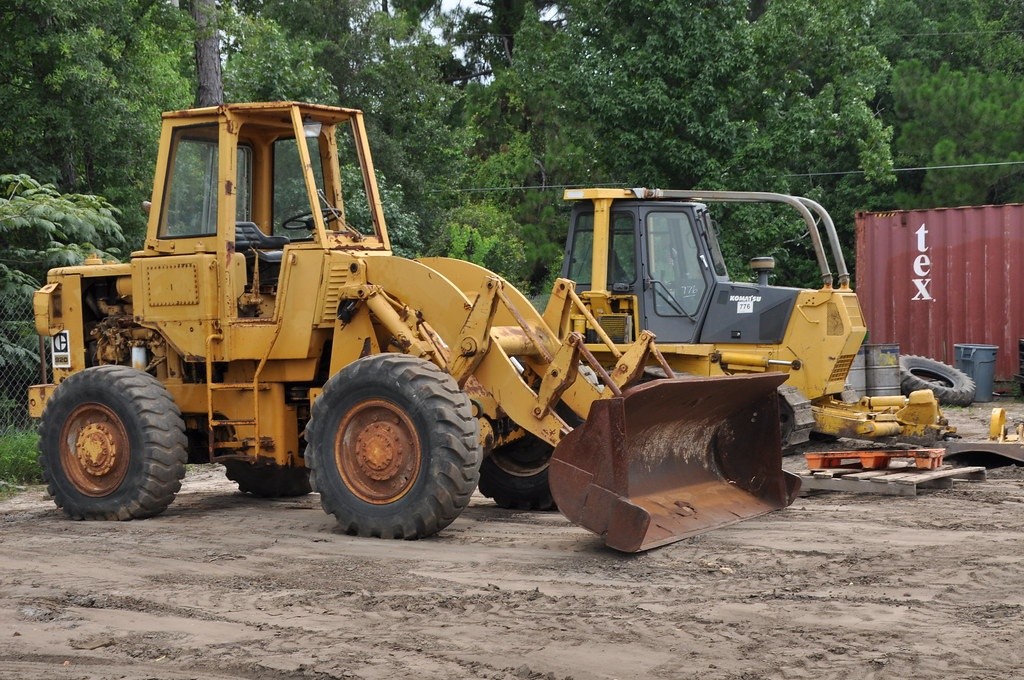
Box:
[953,343,999,403]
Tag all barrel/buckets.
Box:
[845,345,866,400]
[953,343,999,403]
[866,342,901,397]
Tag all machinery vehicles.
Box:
[28,96,804,555]
[541,185,1023,499]
